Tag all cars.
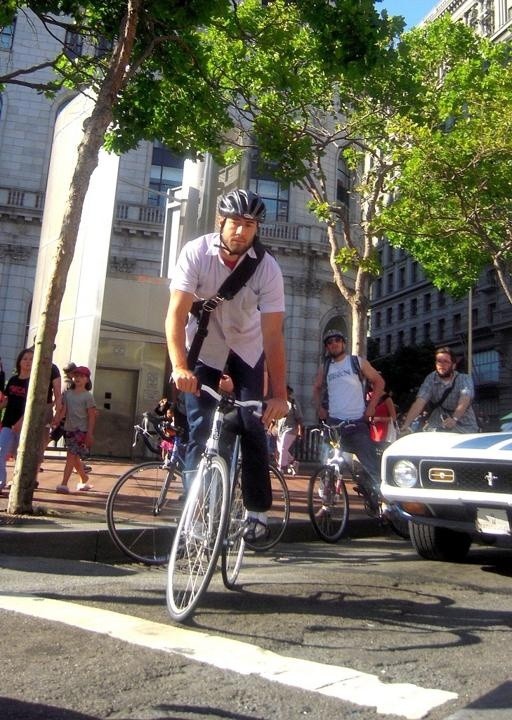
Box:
[377,406,511,570]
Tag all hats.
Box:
[63,362,76,374]
[73,366,90,377]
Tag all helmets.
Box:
[218,189,265,224]
[323,329,347,344]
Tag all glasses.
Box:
[327,336,340,345]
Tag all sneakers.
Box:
[270,461,299,477]
[353,485,370,496]
[316,508,332,519]
[380,501,393,515]
[57,460,92,491]
[242,508,269,542]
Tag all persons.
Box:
[1,336,97,498]
[164,187,290,542]
[153,328,481,519]
